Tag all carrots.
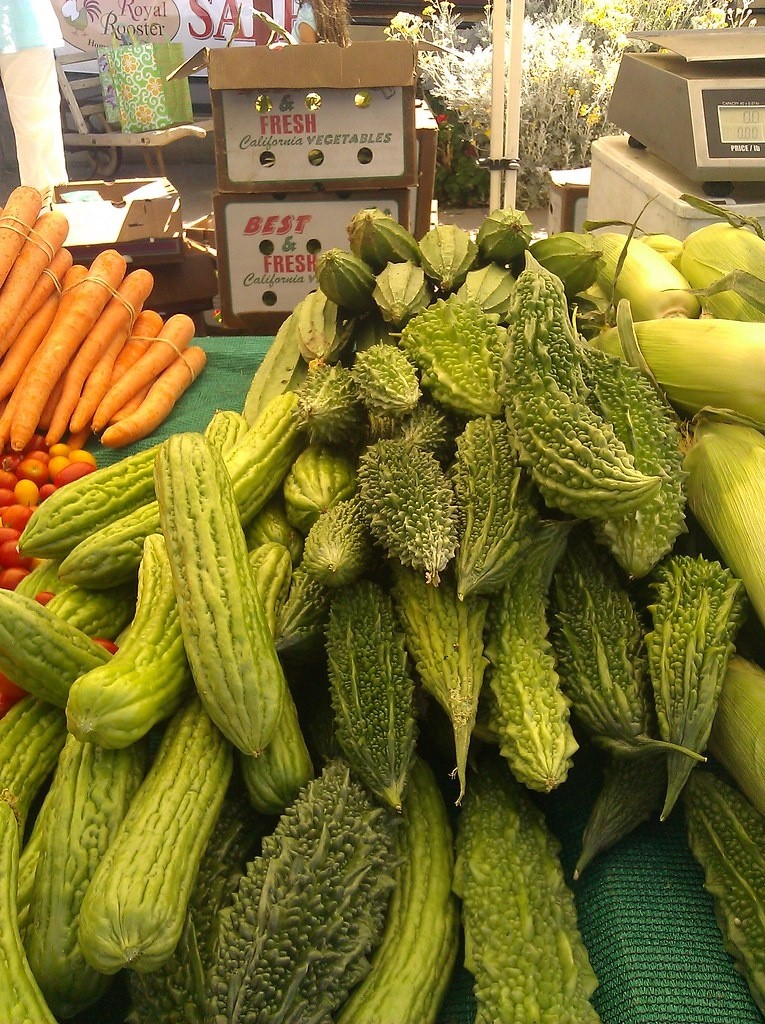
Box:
[0,185,206,451]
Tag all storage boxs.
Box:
[166,41,466,193]
[73,249,219,309]
[413,99,440,244]
[214,189,411,329]
[37,177,185,266]
[548,166,592,238]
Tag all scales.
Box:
[605,27,765,197]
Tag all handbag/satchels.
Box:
[97,23,193,133]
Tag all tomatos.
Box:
[0,433,119,721]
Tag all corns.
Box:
[571,216,765,826]
